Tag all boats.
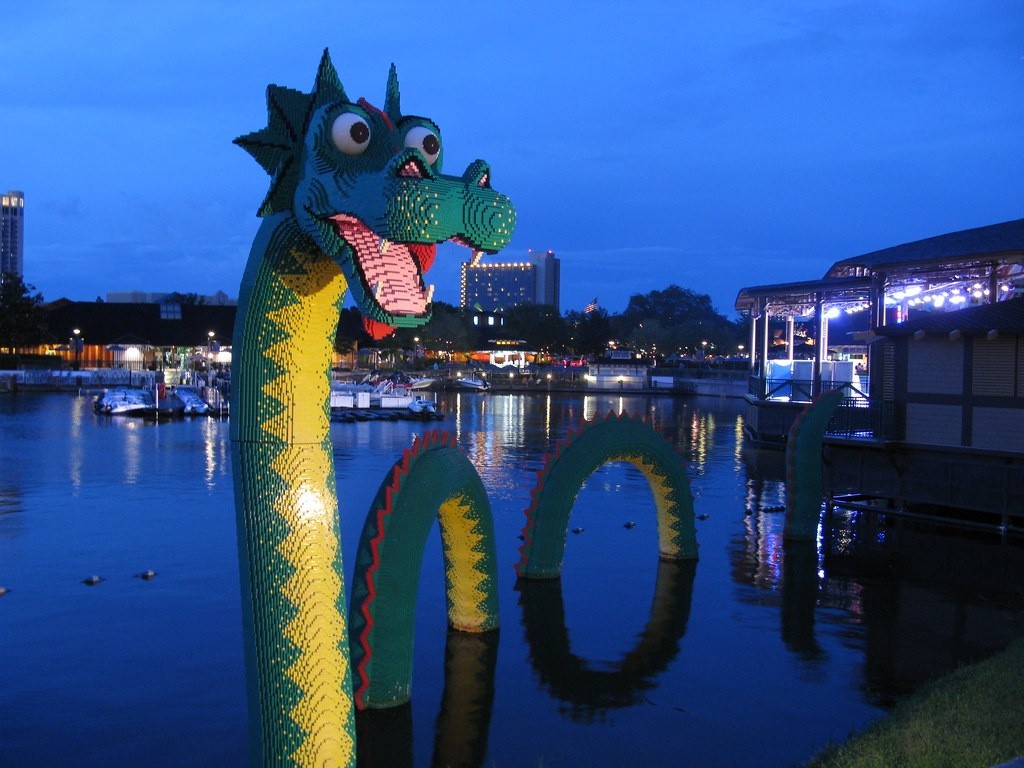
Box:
[91,387,154,414]
[175,389,209,414]
[456,377,492,390]
[142,389,185,415]
[407,399,436,415]
[200,386,229,415]
[371,376,436,389]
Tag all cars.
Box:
[569,355,591,366]
[551,356,570,369]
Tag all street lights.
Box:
[738,343,746,358]
[702,340,707,360]
[208,329,215,388]
[73,326,80,370]
